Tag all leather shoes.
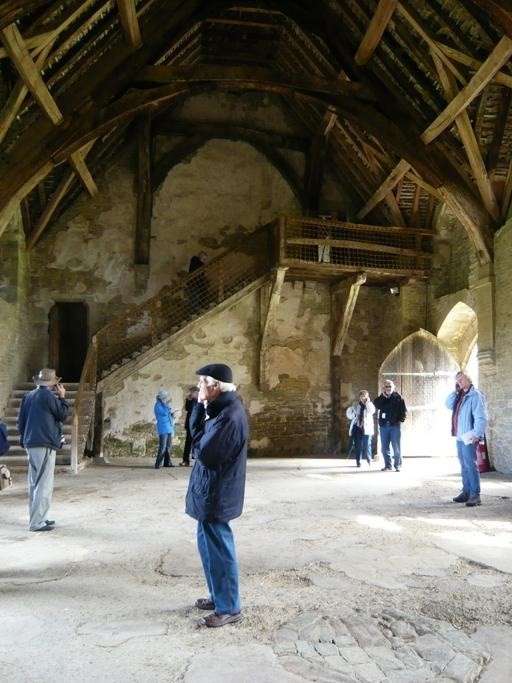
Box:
[34,519,55,532]
[197,611,244,628]
[196,598,215,610]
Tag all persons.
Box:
[153,390,177,469]
[345,389,377,467]
[188,251,209,306]
[0,418,10,457]
[179,385,205,467]
[374,379,408,472]
[17,368,72,532]
[444,370,490,507]
[184,362,251,626]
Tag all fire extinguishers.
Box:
[475,435,490,471]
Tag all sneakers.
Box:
[466,502,481,507]
[356,461,402,472]
[452,491,469,503]
[154,461,189,469]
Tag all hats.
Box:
[189,386,200,392]
[31,367,63,387]
[195,363,234,384]
